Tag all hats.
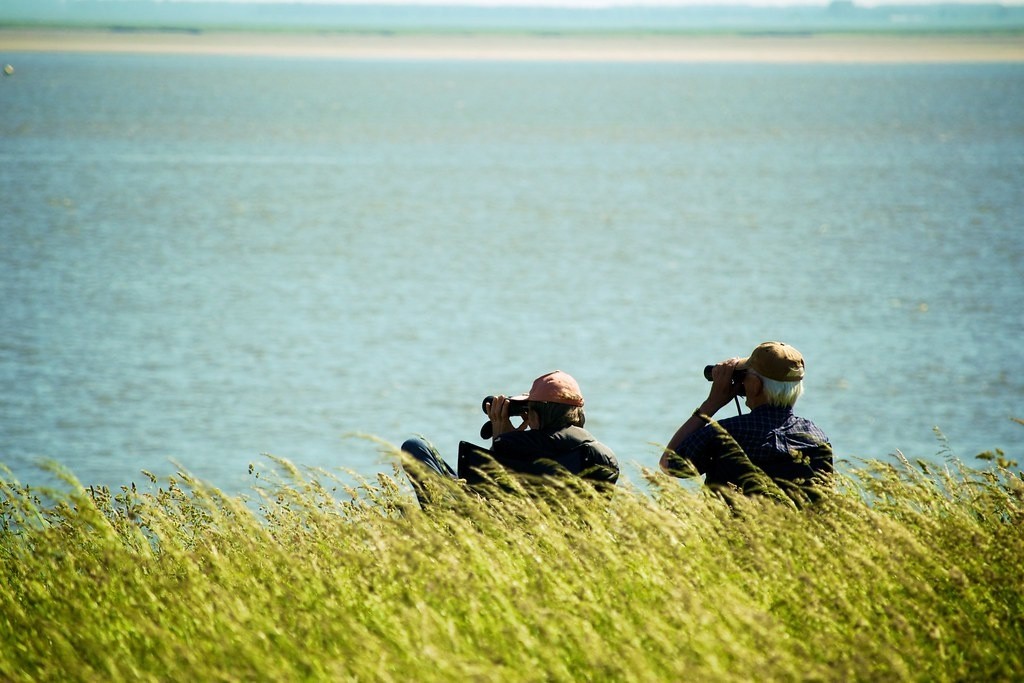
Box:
[735,341,805,381]
[509,370,584,407]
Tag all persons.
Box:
[656,341,835,519]
[400,368,622,517]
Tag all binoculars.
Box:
[482,396,528,417]
[704,365,746,381]
